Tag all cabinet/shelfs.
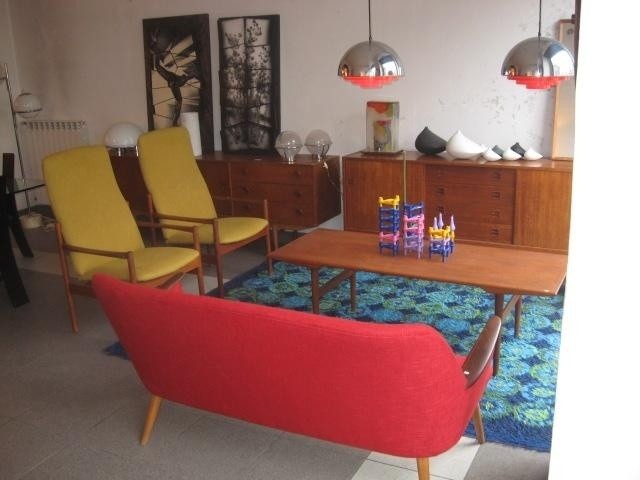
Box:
[415,149,573,255]
[193,150,343,250]
[341,148,425,239]
[106,146,157,216]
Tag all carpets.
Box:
[100,258,567,455]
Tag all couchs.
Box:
[90,271,502,480]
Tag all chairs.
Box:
[1,154,34,308]
[40,145,206,334]
[136,126,272,299]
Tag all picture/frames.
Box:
[216,14,281,152]
[142,14,215,154]
[550,19,575,160]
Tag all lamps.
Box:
[338,0,406,89]
[0,61,43,230]
[104,122,142,157]
[304,129,332,161]
[500,0,576,90]
[274,130,303,161]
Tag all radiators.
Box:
[20,119,88,206]
